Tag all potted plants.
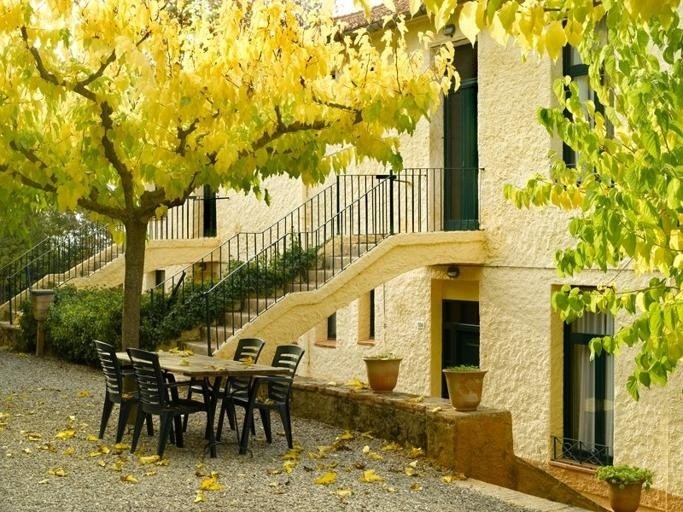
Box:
[443,364,487,411]
[363,353,400,395]
[596,463,656,512]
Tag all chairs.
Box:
[92,336,307,462]
